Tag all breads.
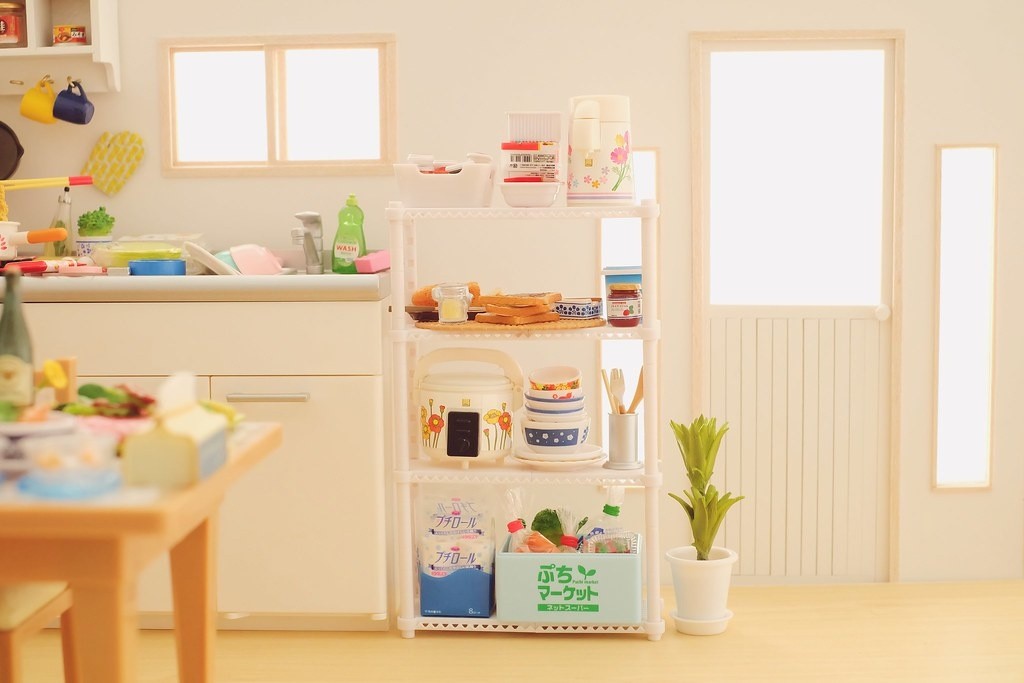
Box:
[411,280,564,325]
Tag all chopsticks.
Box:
[0,177,93,191]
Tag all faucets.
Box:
[289,208,325,275]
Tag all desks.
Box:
[0,405,283,683]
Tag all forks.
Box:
[610,369,625,415]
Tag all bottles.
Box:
[577,503,620,553]
[507,520,534,552]
[432,161,448,174]
[41,187,71,260]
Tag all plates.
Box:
[185,242,236,276]
[513,449,608,467]
[128,260,186,275]
[502,183,560,206]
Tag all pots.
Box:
[0,120,24,181]
[0,221,68,260]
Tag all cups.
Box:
[52,81,95,124]
[431,285,471,322]
[19,80,57,125]
[603,413,645,469]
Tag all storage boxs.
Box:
[495,529,644,626]
[392,161,492,207]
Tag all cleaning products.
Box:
[331,193,369,274]
[42,185,74,261]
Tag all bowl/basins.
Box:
[522,364,590,449]
[215,244,281,276]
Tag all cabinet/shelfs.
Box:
[0,275,393,631]
[1,0,119,97]
[385,200,668,642]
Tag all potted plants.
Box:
[664,414,746,637]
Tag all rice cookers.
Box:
[416,347,519,463]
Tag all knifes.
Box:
[628,367,644,412]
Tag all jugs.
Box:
[566,94,633,205]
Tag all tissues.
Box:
[118,365,235,490]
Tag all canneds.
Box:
[0,2,24,48]
[606,283,643,327]
[431,285,473,325]
[52,24,87,45]
